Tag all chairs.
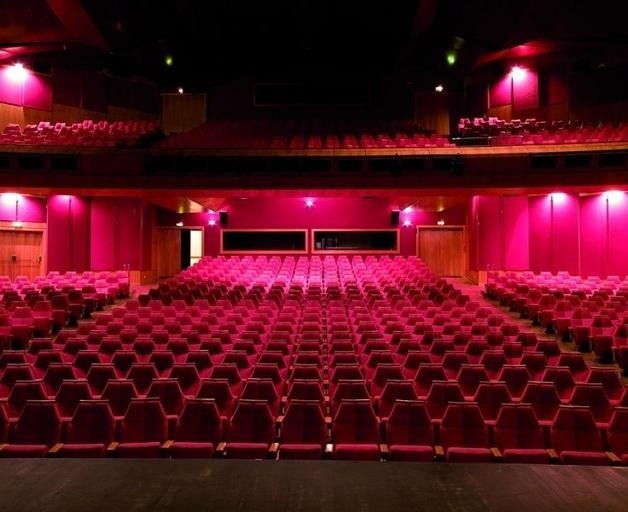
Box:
[457,116,628,146]
[0,120,163,146]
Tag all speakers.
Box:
[220,212,227,224]
[391,210,399,225]
